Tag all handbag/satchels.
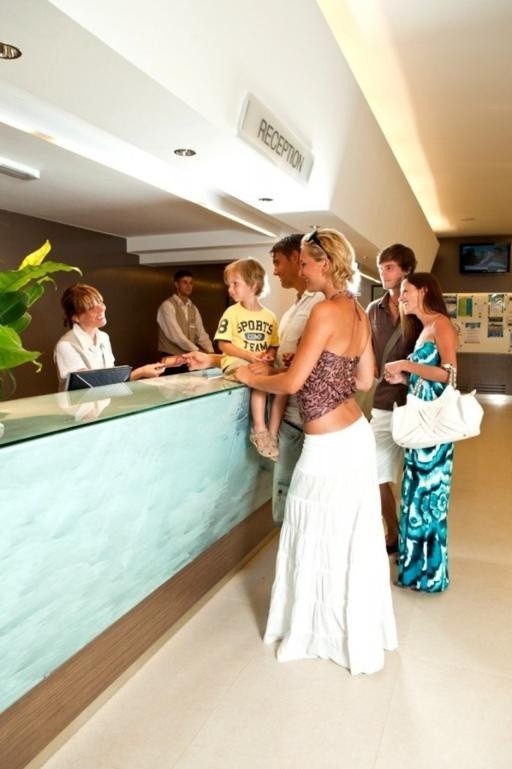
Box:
[390,365,484,448]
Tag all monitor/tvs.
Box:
[64,364,133,391]
[459,241,510,274]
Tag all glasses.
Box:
[306,229,332,260]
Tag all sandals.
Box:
[249,423,280,464]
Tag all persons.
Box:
[178,232,327,526]
[51,284,166,392]
[383,269,458,592]
[212,255,288,464]
[158,267,216,375]
[222,224,403,677]
[52,390,112,423]
[365,241,418,556]
